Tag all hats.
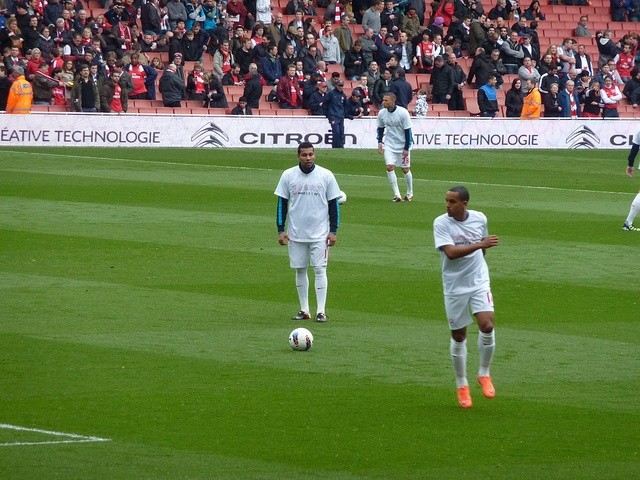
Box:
[351,89,363,97]
[318,81,329,88]
[336,79,345,87]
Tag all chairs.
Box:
[35,1,640,118]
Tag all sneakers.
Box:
[291,310,313,321]
[456,385,474,409]
[621,221,640,233]
[476,373,497,399]
[315,312,330,323]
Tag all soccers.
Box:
[288,327,314,351]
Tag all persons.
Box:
[610,0,640,21]
[321,0,351,90]
[322,81,349,148]
[433,186,498,408]
[275,142,342,322]
[349,1,540,120]
[590,29,640,118]
[376,93,414,203]
[575,16,590,36]
[0,0,328,117]
[621,127,640,231]
[540,39,591,120]
